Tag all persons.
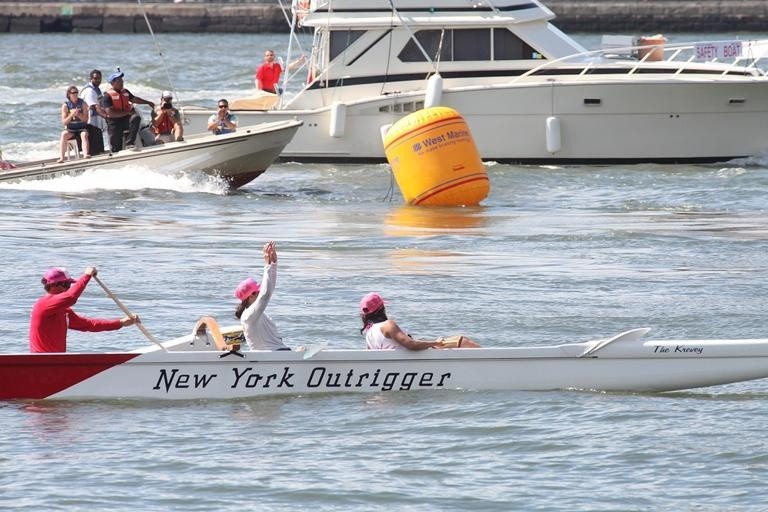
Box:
[255,50,307,94]
[80,70,106,134]
[57,86,91,163]
[29,265,142,353]
[152,91,183,144]
[234,240,292,350]
[103,72,155,153]
[207,99,237,135]
[360,292,481,351]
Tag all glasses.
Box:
[93,77,100,80]
[59,281,71,288]
[70,92,78,94]
[219,105,228,108]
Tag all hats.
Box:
[360,293,390,314]
[162,91,173,98]
[43,267,77,286]
[109,71,124,83]
[235,278,262,301]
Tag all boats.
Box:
[1,117,304,191]
[136,0,768,165]
[1,333,768,402]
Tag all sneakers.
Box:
[178,136,184,140]
[156,139,164,144]
